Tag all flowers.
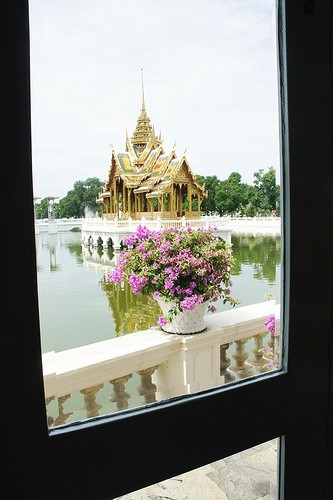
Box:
[108,225,240,326]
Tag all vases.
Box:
[154,296,209,334]
